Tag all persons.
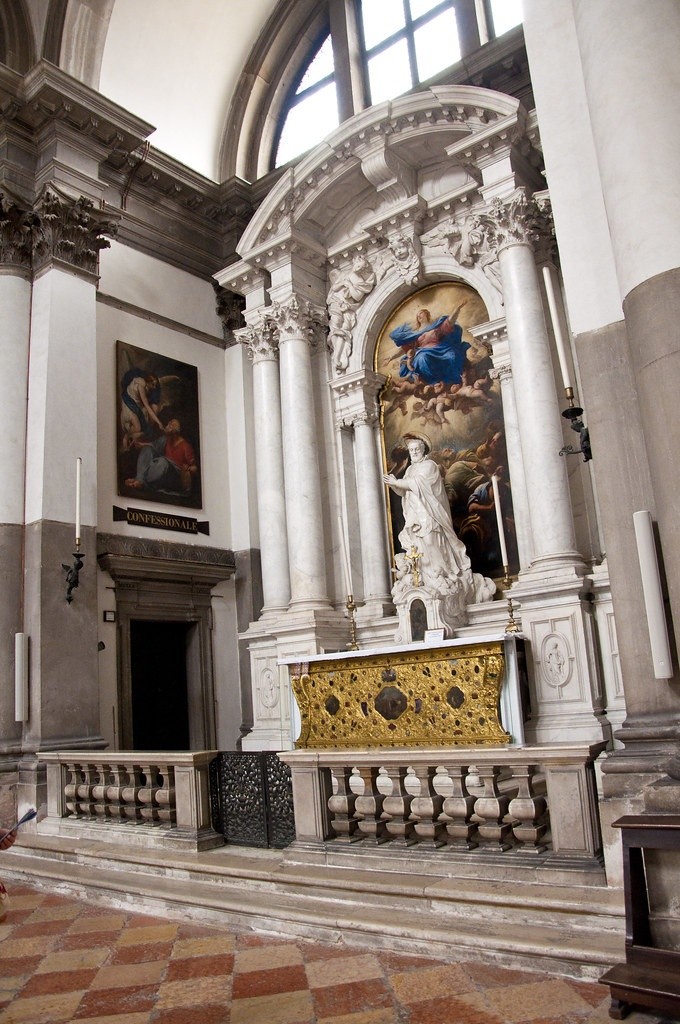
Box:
[325,256,375,372]
[120,368,198,498]
[381,433,495,636]
[388,235,419,280]
[392,297,478,428]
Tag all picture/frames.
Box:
[117,340,202,509]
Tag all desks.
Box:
[279,632,528,745]
[598,815,680,1024]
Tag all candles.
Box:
[337,516,352,596]
[542,266,571,389]
[76,457,81,539]
[491,476,508,566]
